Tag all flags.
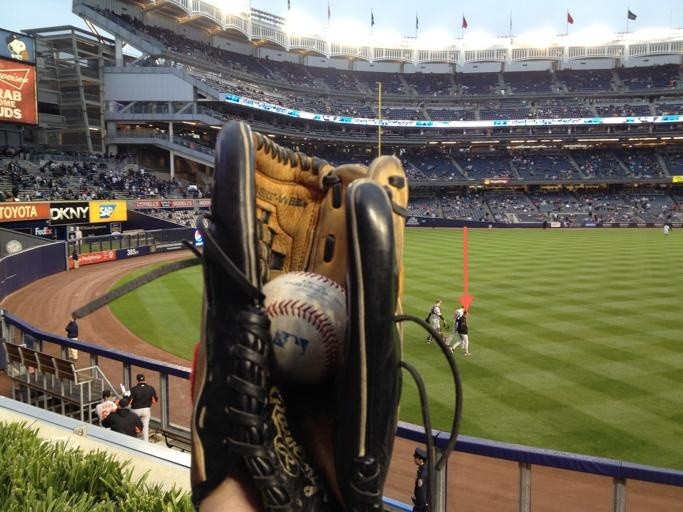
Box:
[415,15,418,30]
[626,10,637,21]
[327,1,330,20]
[370,8,374,26]
[460,16,468,30]
[566,13,574,25]
[287,0,290,10]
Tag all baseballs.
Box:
[263,271,349,377]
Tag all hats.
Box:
[414,448,426,460]
[136,374,145,382]
[463,312,469,315]
[119,399,126,406]
[103,390,110,398]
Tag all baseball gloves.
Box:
[188,118,409,512]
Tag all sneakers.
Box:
[464,352,472,355]
[426,339,432,345]
[449,346,454,354]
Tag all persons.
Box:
[449,308,471,356]
[71,247,80,272]
[119,372,158,442]
[444,305,465,349]
[0,147,212,228]
[540,218,548,229]
[101,400,143,437]
[93,389,119,428]
[64,311,78,362]
[662,222,672,236]
[424,299,449,344]
